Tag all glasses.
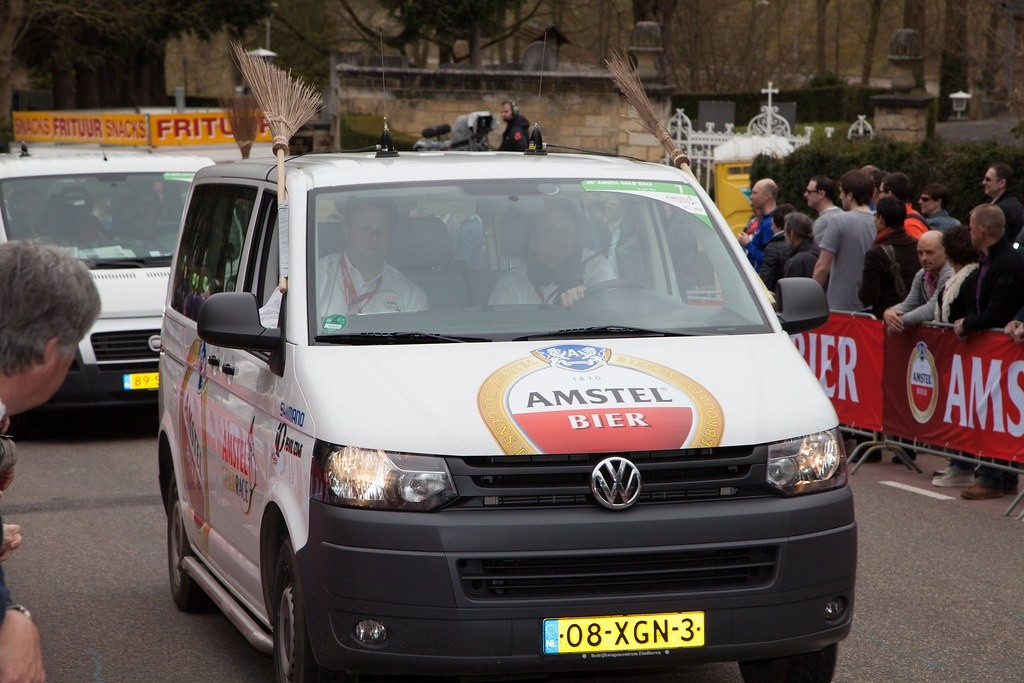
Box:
[920,196,934,201]
[984,175,999,183]
[806,189,819,194]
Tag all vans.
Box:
[0,148,243,432]
[157,151,860,683]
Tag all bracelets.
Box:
[6,604,31,617]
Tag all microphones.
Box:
[422,124,451,138]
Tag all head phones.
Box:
[508,100,520,117]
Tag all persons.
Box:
[488,199,613,307]
[183,287,204,321]
[0,241,102,683]
[738,162,1024,500]
[259,197,429,328]
[498,100,531,152]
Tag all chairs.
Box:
[386,217,463,313]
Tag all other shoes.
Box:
[932,470,975,487]
[891,449,917,463]
[934,466,960,477]
[850,448,881,463]
[961,481,1003,499]
[998,483,1019,496]
